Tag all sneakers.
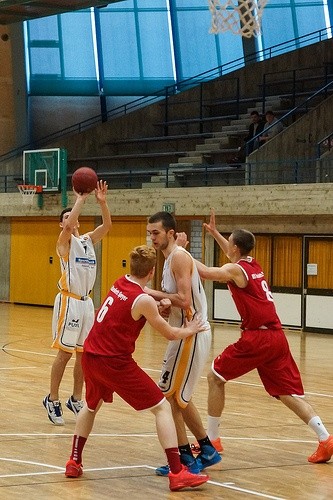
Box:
[209,438,223,453]
[168,463,210,488]
[66,394,84,413]
[194,446,222,467]
[190,436,224,455]
[42,394,65,426]
[155,454,201,476]
[65,460,84,479]
[307,434,333,466]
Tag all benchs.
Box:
[12,62,333,191]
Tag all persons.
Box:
[66,245,210,491]
[176,207,333,463]
[43,180,112,421]
[231,110,284,164]
[145,210,223,477]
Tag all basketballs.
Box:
[72,166,98,196]
